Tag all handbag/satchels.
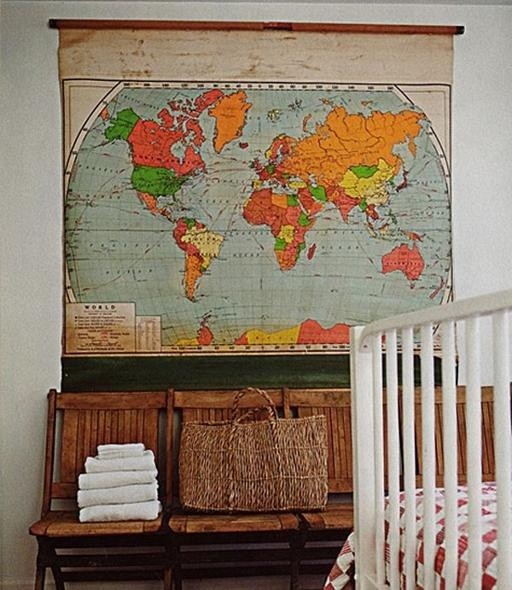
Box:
[178,386,330,513]
